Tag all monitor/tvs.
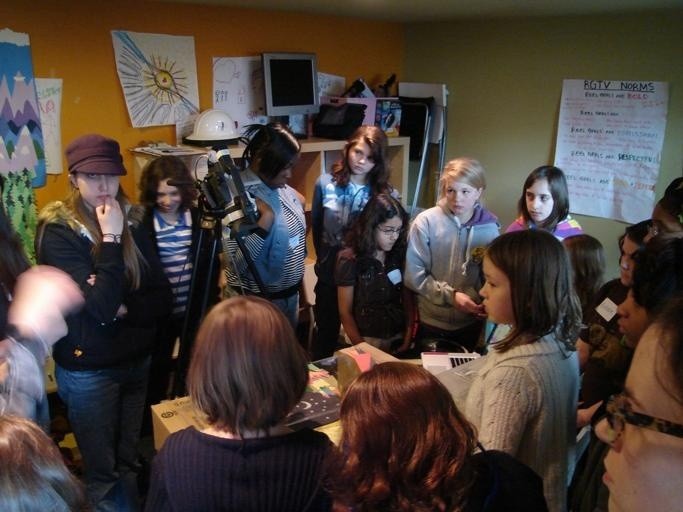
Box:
[262,53,320,116]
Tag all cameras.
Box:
[167,142,263,240]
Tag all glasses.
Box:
[377,225,403,236]
[607,395,683,446]
[647,221,683,238]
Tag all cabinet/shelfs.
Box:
[134,135,410,264]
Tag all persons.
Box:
[574,220,661,375]
[594,296,681,510]
[147,294,337,511]
[652,177,683,240]
[34,133,170,504]
[221,123,311,332]
[462,230,580,511]
[403,156,500,350]
[126,157,210,436]
[0,202,83,512]
[335,192,414,357]
[483,164,582,353]
[327,362,546,511]
[577,234,682,429]
[312,125,399,356]
[559,235,604,308]
[0,413,83,507]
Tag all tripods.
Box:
[170,215,315,400]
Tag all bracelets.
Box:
[100,233,122,243]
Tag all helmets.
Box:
[185,110,241,140]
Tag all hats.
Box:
[65,134,127,175]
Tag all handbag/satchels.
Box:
[314,103,366,139]
